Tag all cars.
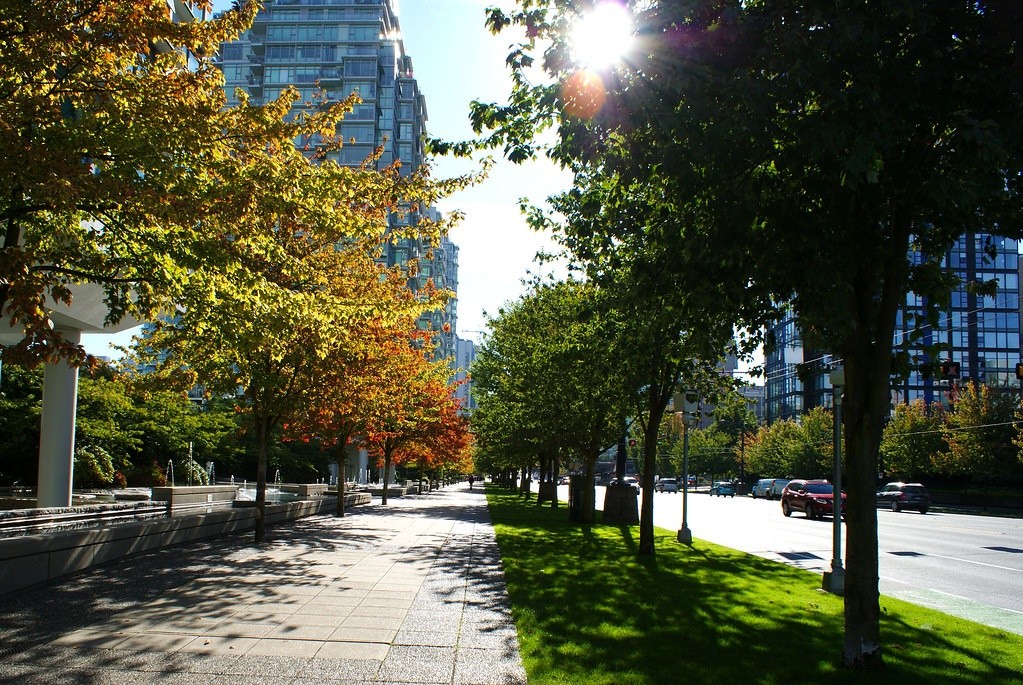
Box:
[473,474,495,484]
[680,474,697,485]
[654,477,677,494]
[709,481,734,498]
[610,474,662,496]
[515,472,571,486]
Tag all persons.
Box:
[469,474,474,490]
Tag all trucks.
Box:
[752,478,788,499]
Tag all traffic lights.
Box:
[628,440,636,447]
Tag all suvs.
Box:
[877,481,932,514]
[780,478,848,521]
